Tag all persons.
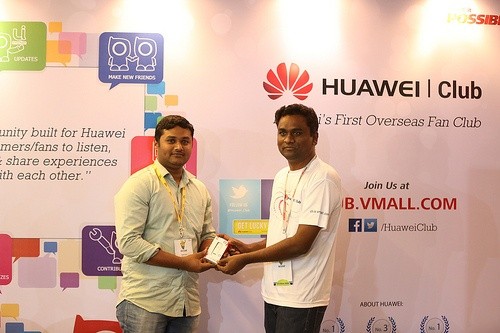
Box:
[214,103,343,333]
[111,114,237,333]
